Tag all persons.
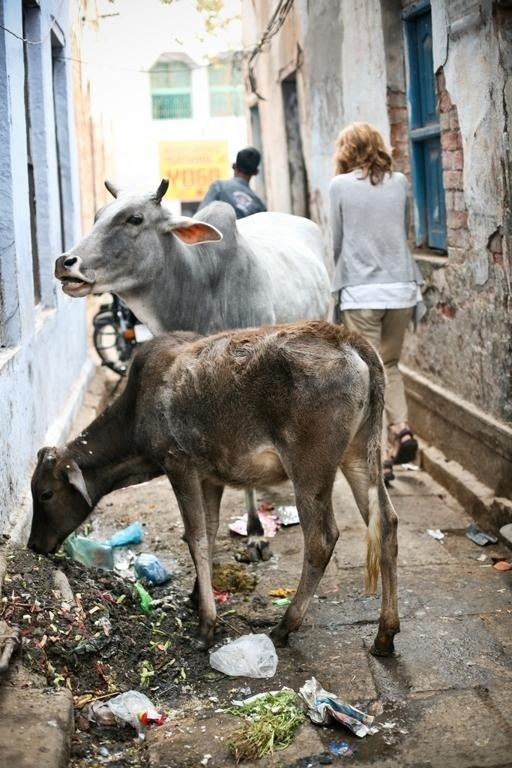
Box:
[190,148,265,217]
[328,125,420,490]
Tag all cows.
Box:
[53,178,335,564]
[26,316,402,662]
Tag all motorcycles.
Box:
[92,286,152,384]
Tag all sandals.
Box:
[383,460,394,481]
[392,429,417,464]
